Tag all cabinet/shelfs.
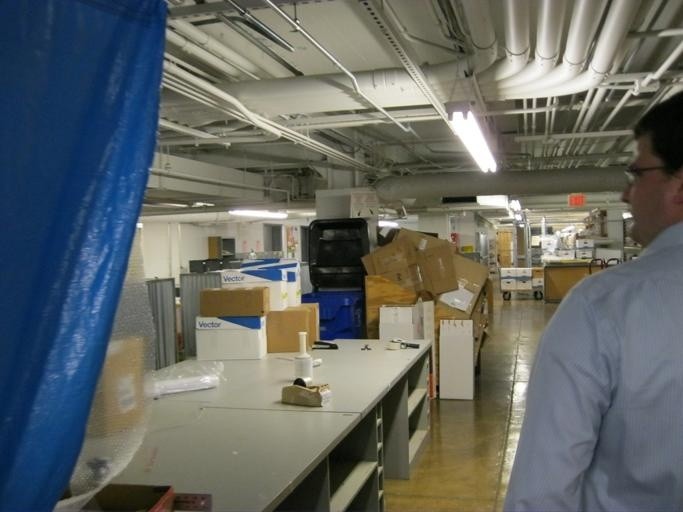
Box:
[450,206,646,303]
[111,338,433,512]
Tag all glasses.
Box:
[625,166,664,184]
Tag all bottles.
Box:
[293,332,314,384]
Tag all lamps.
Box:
[444,100,497,173]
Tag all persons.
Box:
[502,87,683,512]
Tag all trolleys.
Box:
[492,254,543,300]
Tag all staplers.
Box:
[312,340,339,350]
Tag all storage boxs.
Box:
[85,334,144,434]
[78,483,175,512]
[179,187,490,400]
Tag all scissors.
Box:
[361,344,371,351]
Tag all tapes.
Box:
[292,376,315,389]
[387,340,401,349]
